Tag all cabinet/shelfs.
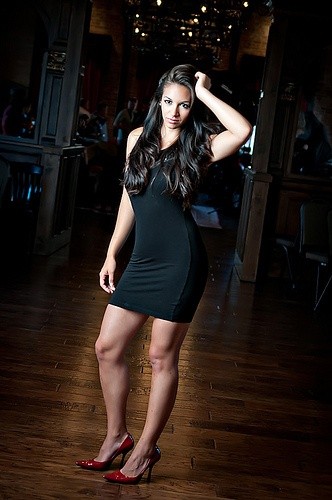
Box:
[268,190,332,279]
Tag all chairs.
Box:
[299,198,332,312]
[0,153,42,288]
